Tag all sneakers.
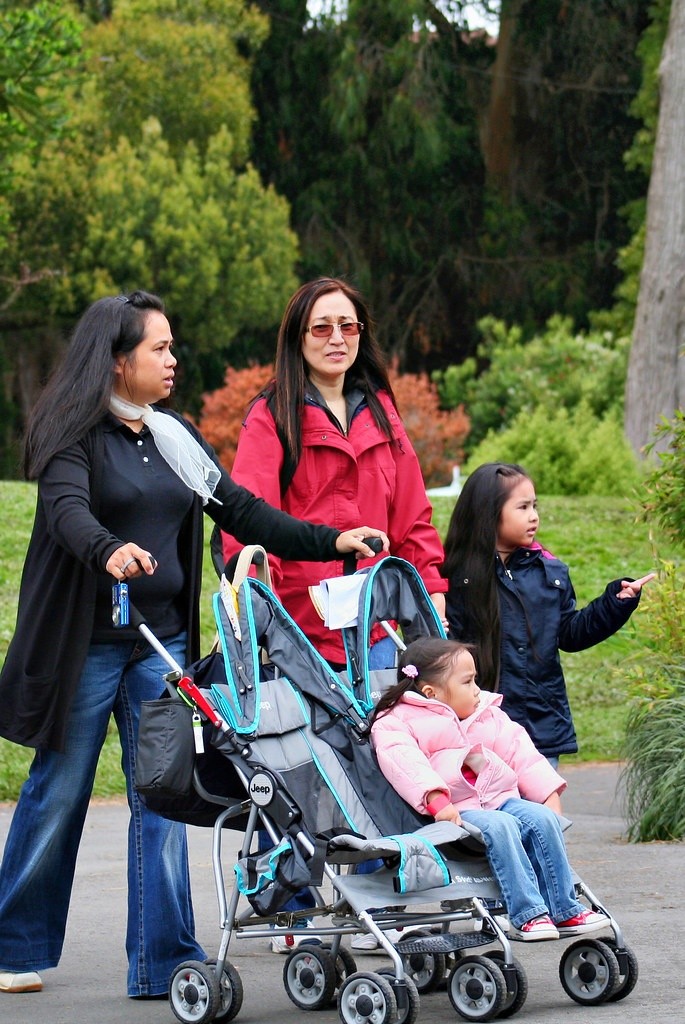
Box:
[508,914,560,941]
[555,908,611,935]
[350,922,430,950]
[0,970,43,993]
[269,918,322,954]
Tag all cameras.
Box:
[112,583,129,630]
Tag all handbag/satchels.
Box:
[135,545,286,832]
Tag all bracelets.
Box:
[426,793,450,816]
[441,618,450,632]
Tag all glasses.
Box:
[304,322,364,338]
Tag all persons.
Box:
[0,289,391,999]
[439,463,655,772]
[368,637,611,942]
[209,276,450,954]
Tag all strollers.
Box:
[128,537,640,1024]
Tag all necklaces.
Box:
[325,400,347,434]
[498,551,512,553]
